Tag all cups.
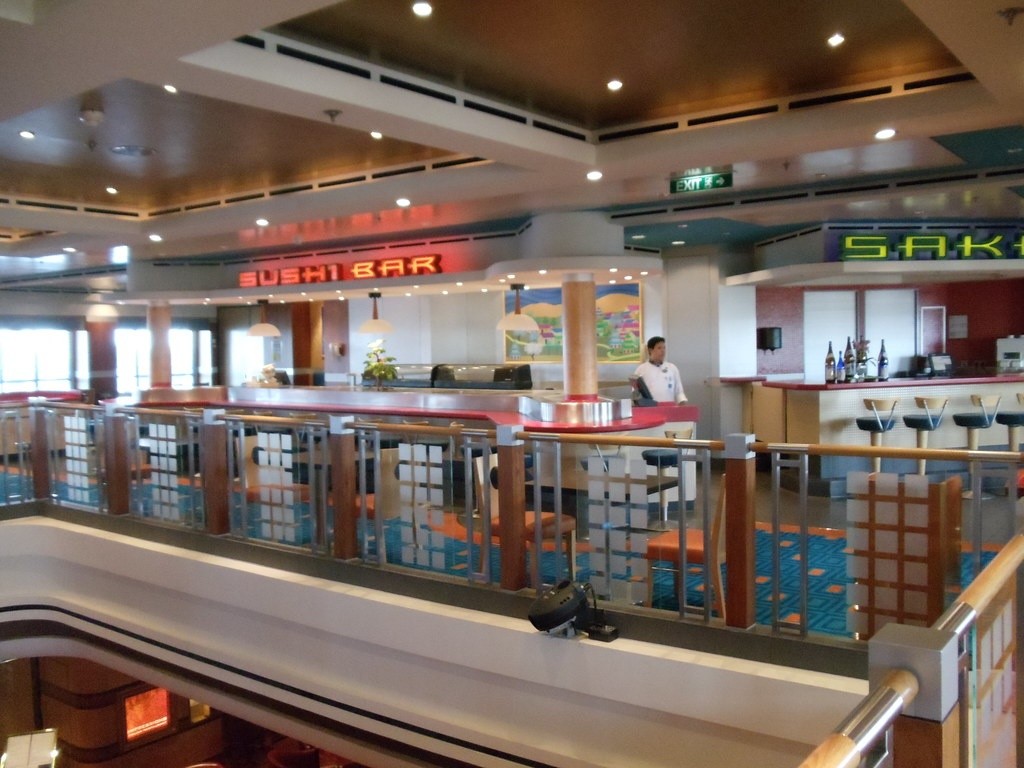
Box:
[347,375,355,385]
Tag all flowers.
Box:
[851,335,876,364]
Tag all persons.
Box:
[632,337,688,405]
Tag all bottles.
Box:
[924,357,931,374]
[844,336,855,383]
[825,341,835,383]
[837,350,845,384]
[878,339,889,381]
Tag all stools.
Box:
[995,393,1024,498]
[181,407,497,519]
[952,394,1003,500]
[580,431,630,529]
[903,397,949,476]
[641,428,694,532]
[856,397,901,471]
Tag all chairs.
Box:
[474,453,577,588]
[641,473,727,620]
[355,446,421,566]
[94,421,311,552]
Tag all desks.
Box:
[266,447,380,551]
[129,387,701,522]
[525,468,678,601]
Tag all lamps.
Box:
[246,298,282,336]
[496,282,540,330]
[357,292,396,334]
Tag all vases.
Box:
[856,361,868,379]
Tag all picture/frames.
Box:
[503,280,644,365]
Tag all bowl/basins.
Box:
[28,396,46,403]
[1008,334,1014,339]
